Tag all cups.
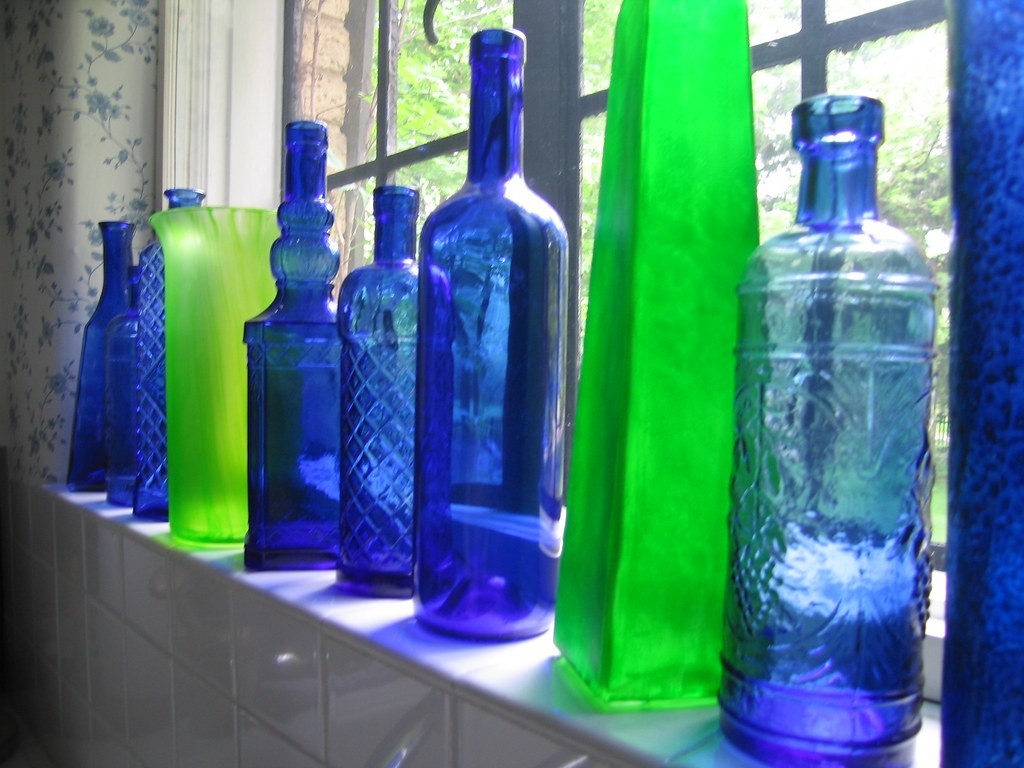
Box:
[150,207,280,552]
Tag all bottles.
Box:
[66,220,137,492]
[106,264,139,507]
[555,0,752,710]
[412,27,570,641]
[723,93,934,768]
[133,188,206,527]
[243,119,340,571]
[336,185,419,600]
